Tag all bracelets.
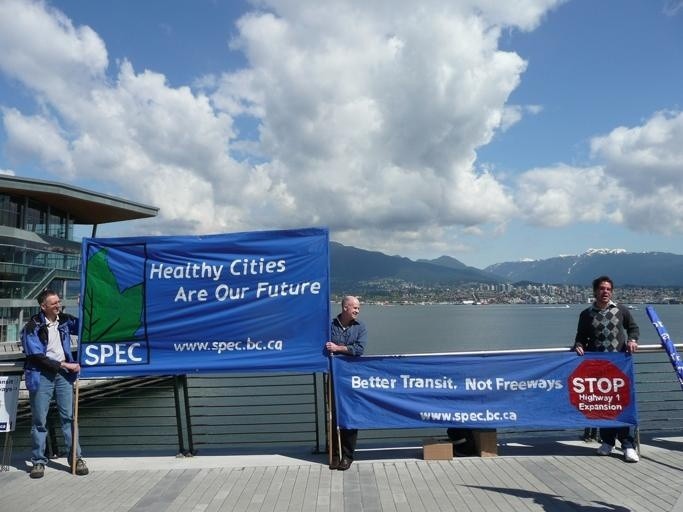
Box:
[628,339,636,343]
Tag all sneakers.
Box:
[30,464,44,478]
[595,443,614,456]
[68,456,87,475]
[624,448,638,462]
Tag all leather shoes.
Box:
[329,458,339,469]
[336,459,352,470]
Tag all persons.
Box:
[22,290,88,478]
[324,295,366,471]
[573,276,640,462]
